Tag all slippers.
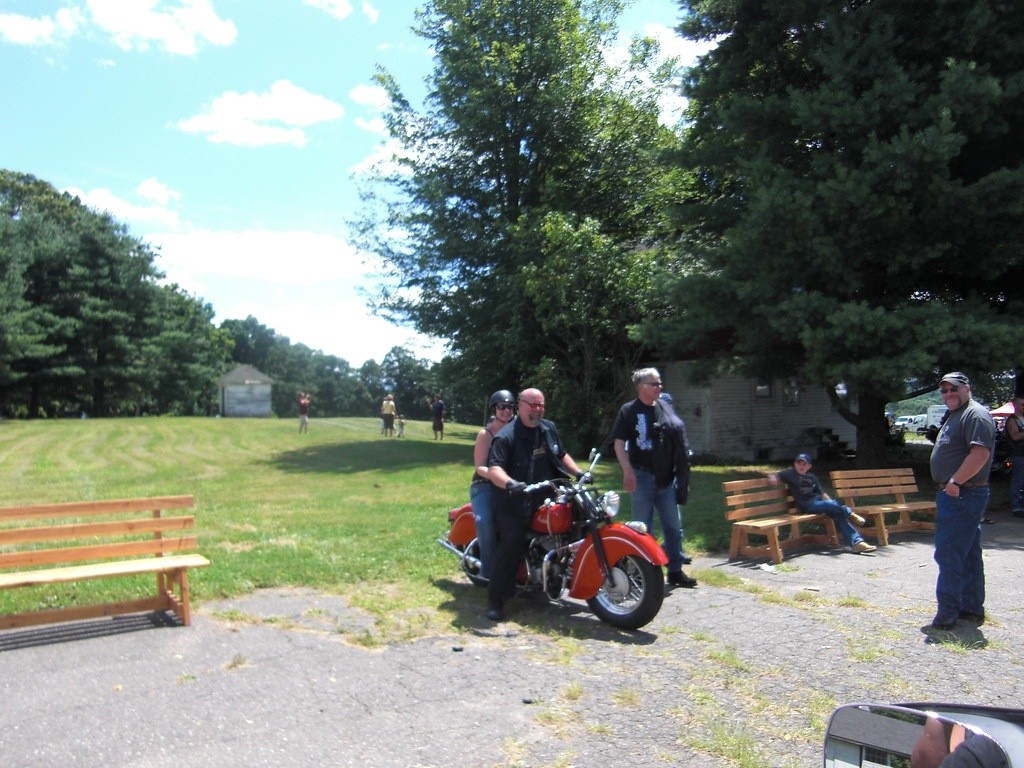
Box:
[982,518,994,525]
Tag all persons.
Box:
[614,368,698,587]
[768,454,877,554]
[380,394,407,438]
[427,394,446,440]
[469,388,594,620]
[1005,395,1024,518]
[930,372,996,630]
[297,391,311,435]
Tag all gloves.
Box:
[506,479,527,496]
[576,470,594,484]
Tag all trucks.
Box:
[909,405,949,435]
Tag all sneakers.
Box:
[848,512,866,526]
[666,570,698,587]
[851,541,877,554]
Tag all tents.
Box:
[989,402,1015,417]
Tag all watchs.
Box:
[949,477,962,486]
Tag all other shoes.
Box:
[680,553,693,564]
[1013,511,1024,519]
[919,624,959,636]
[961,612,986,623]
[485,594,506,621]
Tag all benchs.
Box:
[830,467,937,545]
[721,476,843,564]
[0,494,213,629]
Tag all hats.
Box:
[794,454,811,464]
[939,372,969,386]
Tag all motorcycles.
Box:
[435,444,669,629]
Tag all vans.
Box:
[894,415,914,433]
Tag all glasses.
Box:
[520,399,546,409]
[646,381,663,386]
[496,403,515,410]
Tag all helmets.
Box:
[488,389,515,409]
[939,385,959,394]
